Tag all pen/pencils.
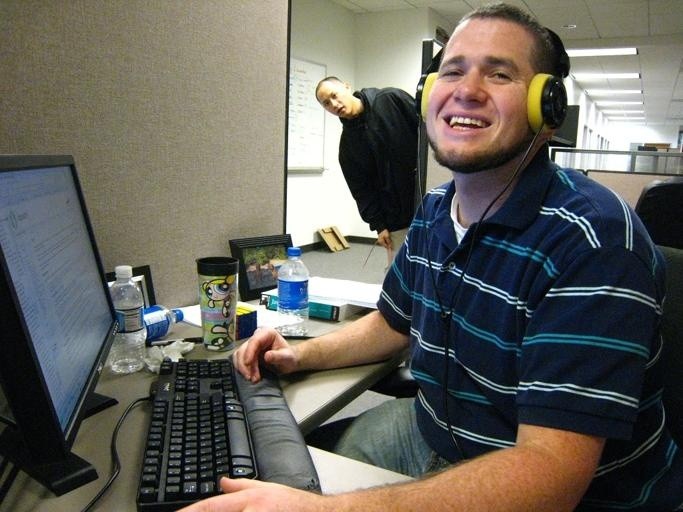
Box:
[149,337,204,346]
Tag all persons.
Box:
[167,0,683,511]
[315,76,424,262]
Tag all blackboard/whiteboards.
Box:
[287,57,328,174]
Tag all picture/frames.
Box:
[229,234,293,302]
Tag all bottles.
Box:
[141,304,184,344]
[277,246,309,340]
[107,264,146,375]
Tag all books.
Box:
[261,290,367,321]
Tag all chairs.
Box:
[635,177,683,455]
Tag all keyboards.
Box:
[136,355,323,511]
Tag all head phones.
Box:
[416,26,571,139]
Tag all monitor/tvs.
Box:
[0,154,120,496]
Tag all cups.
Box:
[196,256,239,352]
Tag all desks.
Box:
[0,273,416,511]
